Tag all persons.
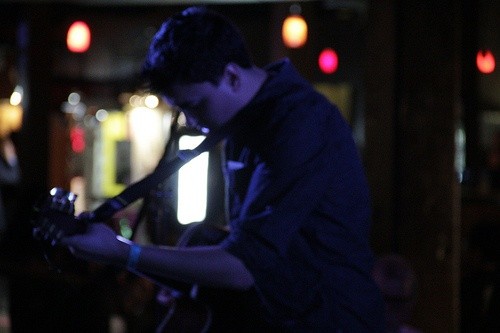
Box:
[475,135,500,196]
[459,221,500,333]
[55,8,393,333]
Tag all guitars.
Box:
[26,185,229,333]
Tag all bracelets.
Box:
[125,242,141,274]
